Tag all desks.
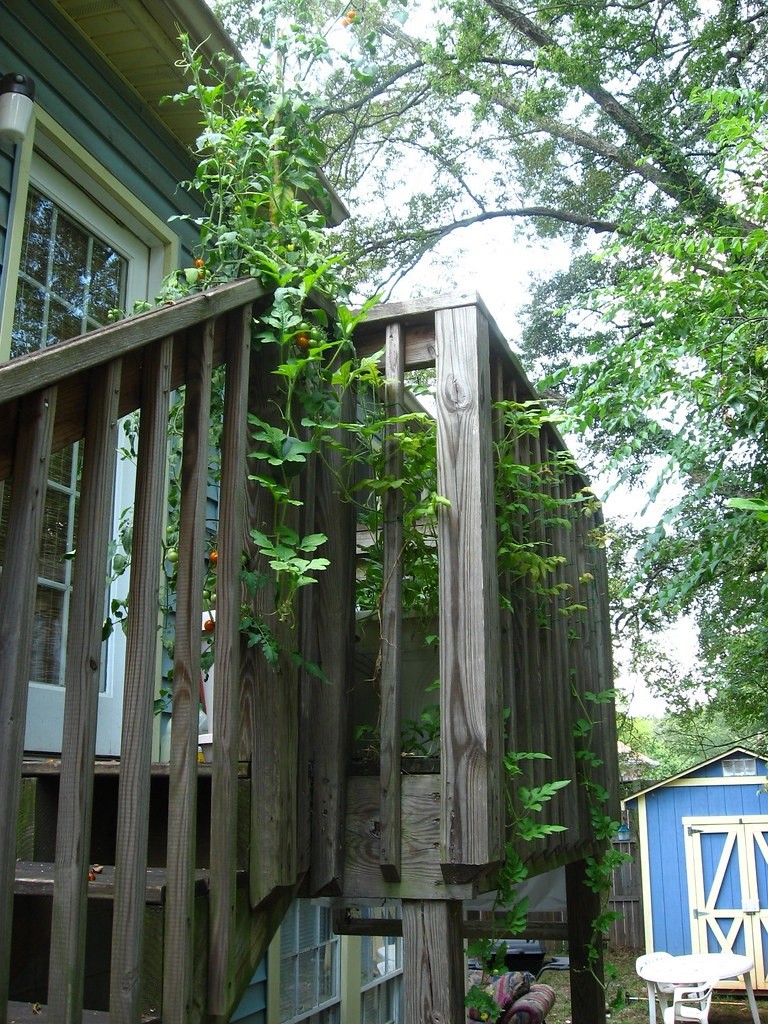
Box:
[640,953,761,1024]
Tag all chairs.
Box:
[662,974,719,1024]
[635,951,705,1018]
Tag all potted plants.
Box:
[356,705,440,774]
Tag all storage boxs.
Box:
[489,940,547,976]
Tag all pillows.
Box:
[468,971,536,1022]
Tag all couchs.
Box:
[467,984,558,1024]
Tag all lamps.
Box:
[0,71,35,144]
[617,824,631,841]
